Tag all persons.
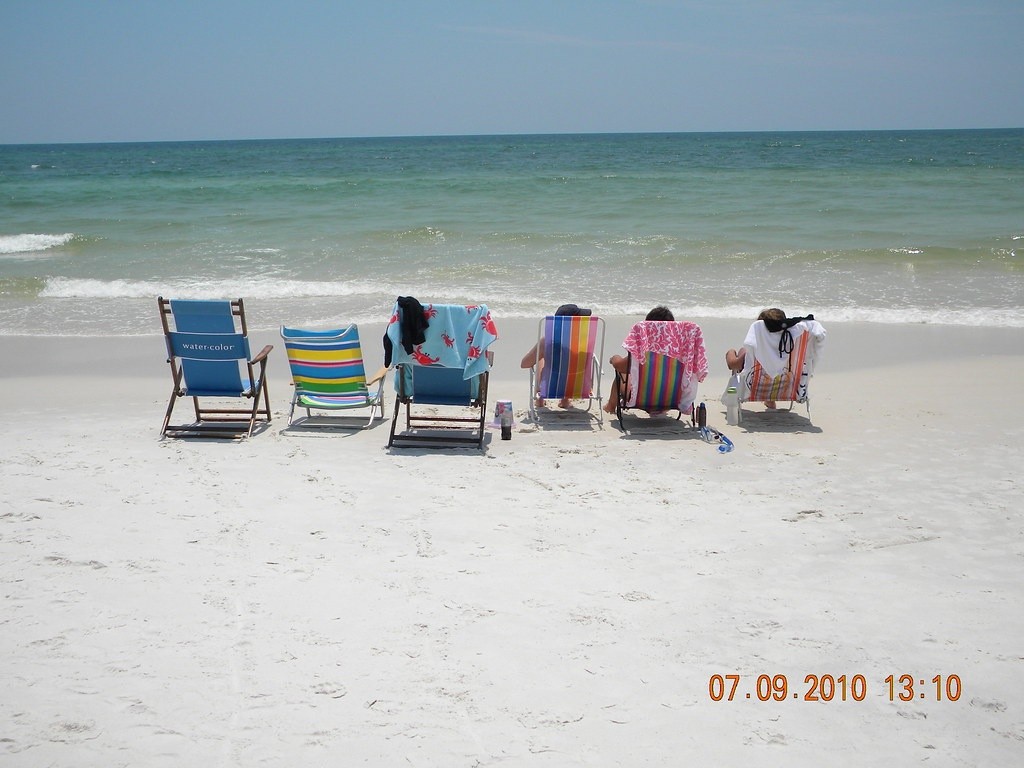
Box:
[726,309,786,409]
[520,304,591,408]
[602,306,674,415]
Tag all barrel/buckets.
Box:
[491,400,516,429]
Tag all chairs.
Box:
[157,296,273,439]
[280,322,389,430]
[614,321,709,435]
[529,315,606,426]
[385,302,500,450]
[721,314,826,426]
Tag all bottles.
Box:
[727,387,738,425]
[699,403,706,430]
[502,408,512,440]
[696,405,700,423]
